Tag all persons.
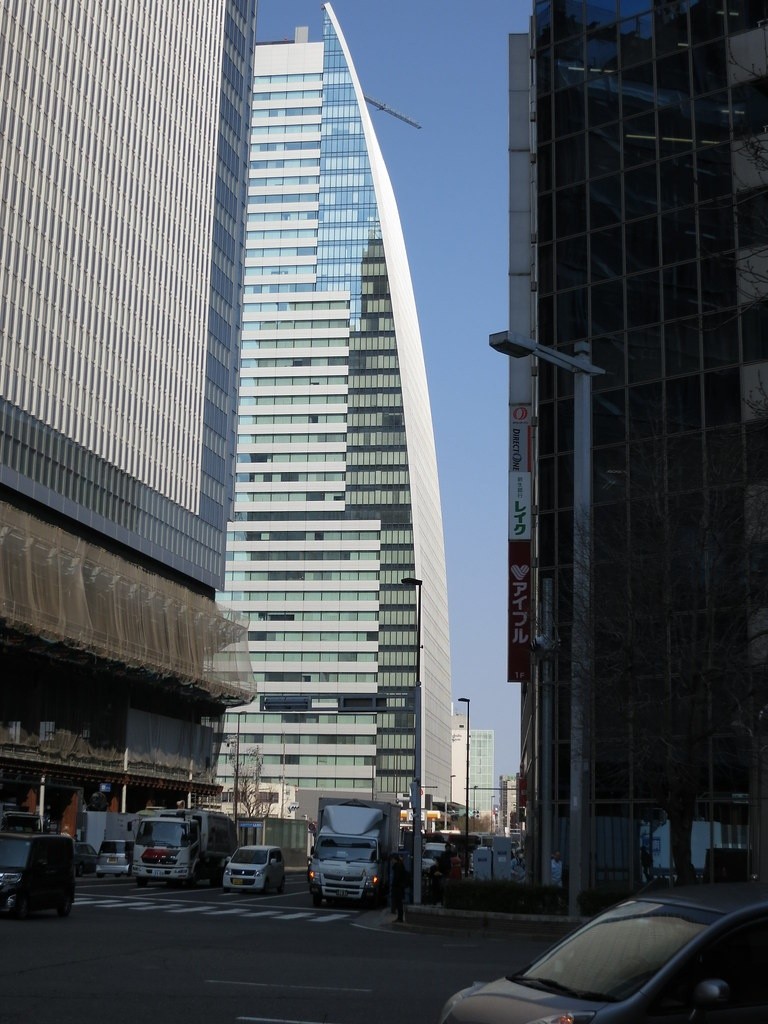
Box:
[639,844,652,885]
[430,844,461,906]
[390,852,408,923]
[550,851,562,888]
[510,842,525,886]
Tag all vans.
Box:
[222,845,286,895]
[0,831,77,921]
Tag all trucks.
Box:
[306,798,401,908]
[127,807,236,888]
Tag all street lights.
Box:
[450,775,456,803]
[401,577,423,834]
[233,711,248,825]
[457,697,470,876]
[491,796,496,832]
[488,331,607,921]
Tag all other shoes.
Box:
[392,918,403,923]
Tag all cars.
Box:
[96,839,133,880]
[74,842,99,878]
[438,879,768,1024]
[404,825,489,879]
